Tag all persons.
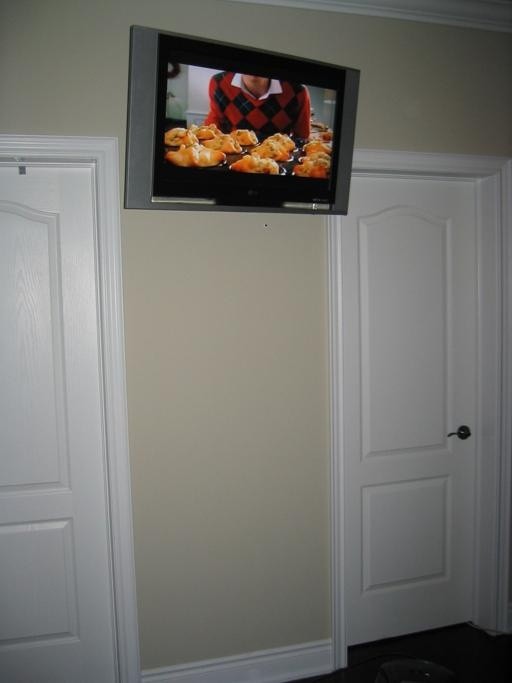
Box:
[199,69,313,143]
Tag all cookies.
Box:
[164,123,333,178]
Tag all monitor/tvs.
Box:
[124,24,360,216]
